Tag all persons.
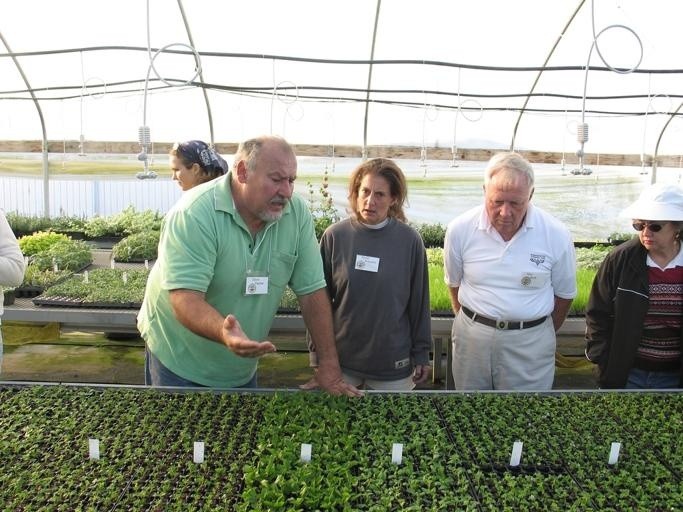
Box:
[170,140,227,191]
[581,185,683,389]
[136,136,363,398]
[0,208,26,376]
[444,152,577,392]
[303,158,432,390]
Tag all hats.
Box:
[623,181,683,224]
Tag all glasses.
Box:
[632,221,668,232]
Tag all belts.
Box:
[461,305,548,335]
